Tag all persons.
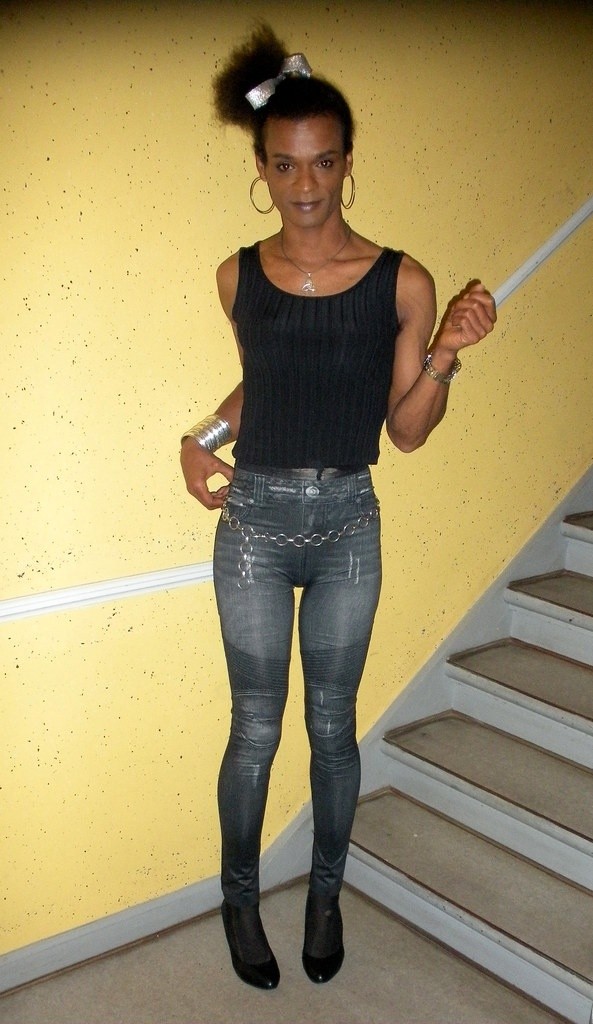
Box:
[181,23,499,989]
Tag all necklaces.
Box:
[281,223,351,291]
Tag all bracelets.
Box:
[181,414,232,452]
[422,349,462,383]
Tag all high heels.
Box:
[301,891,345,984]
[221,900,282,989]
